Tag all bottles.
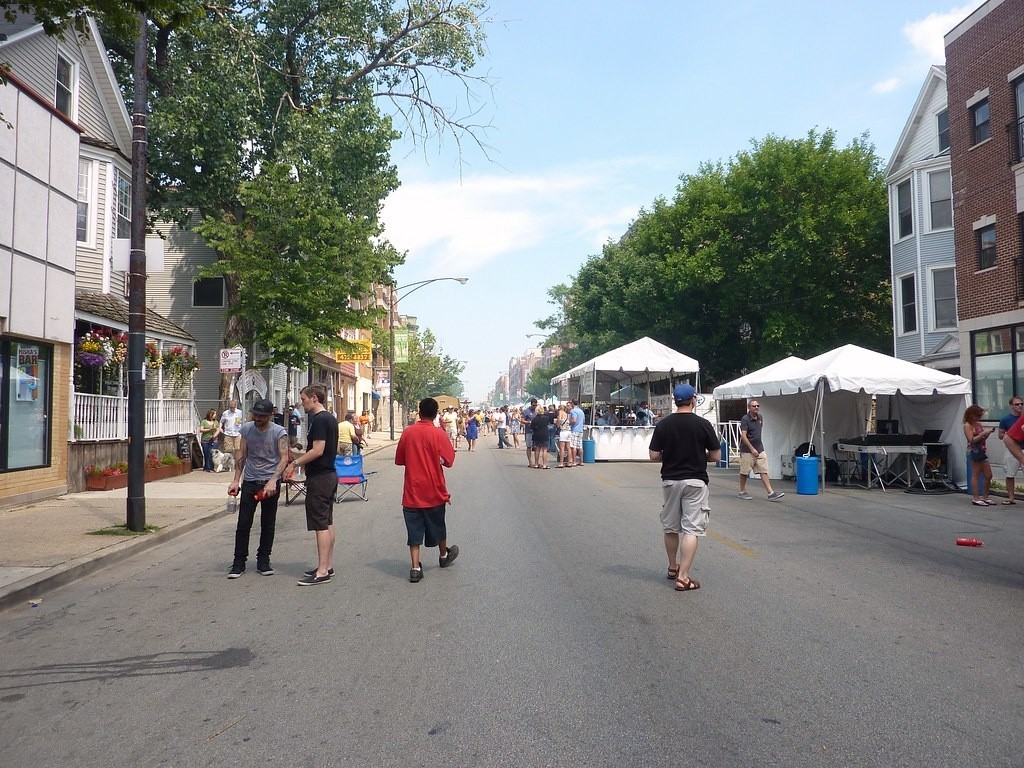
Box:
[838,475,841,485]
[254,488,268,500]
[227,492,237,514]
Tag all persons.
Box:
[963,405,997,506]
[999,397,1024,506]
[282,385,339,586]
[395,398,458,582]
[648,384,721,590]
[737,400,785,500]
[228,399,287,577]
[200,400,375,472]
[407,399,659,468]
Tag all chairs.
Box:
[833,443,862,481]
[334,455,368,503]
[285,452,307,506]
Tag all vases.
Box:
[87,461,192,491]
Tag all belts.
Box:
[254,480,268,485]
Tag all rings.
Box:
[286,474,287,475]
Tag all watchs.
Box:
[292,460,297,468]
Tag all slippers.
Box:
[534,465,543,469]
[571,463,584,467]
[971,499,997,506]
[1002,500,1016,505]
[543,466,551,469]
[555,464,572,468]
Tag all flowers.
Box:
[76,327,199,397]
[84,453,181,477]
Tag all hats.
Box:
[250,399,273,415]
[642,401,648,405]
[449,405,454,409]
[289,407,294,411]
[673,385,698,401]
[345,414,352,420]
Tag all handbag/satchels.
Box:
[972,451,988,462]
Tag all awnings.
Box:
[372,389,381,399]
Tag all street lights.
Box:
[389,276,469,441]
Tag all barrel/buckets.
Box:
[796,454,818,494]
[716,442,730,468]
[583,440,595,462]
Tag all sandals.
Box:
[675,577,700,590]
[667,564,680,579]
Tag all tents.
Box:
[713,344,969,493]
[550,337,699,425]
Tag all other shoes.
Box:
[528,464,540,468]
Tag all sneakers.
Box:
[304,568,335,577]
[297,574,332,585]
[227,563,247,578]
[768,491,785,500]
[737,491,752,500]
[439,545,459,567]
[256,561,274,575]
[410,562,423,582]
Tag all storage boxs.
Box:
[781,454,822,481]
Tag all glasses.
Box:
[752,405,760,407]
[533,405,537,407]
[1013,403,1023,406]
